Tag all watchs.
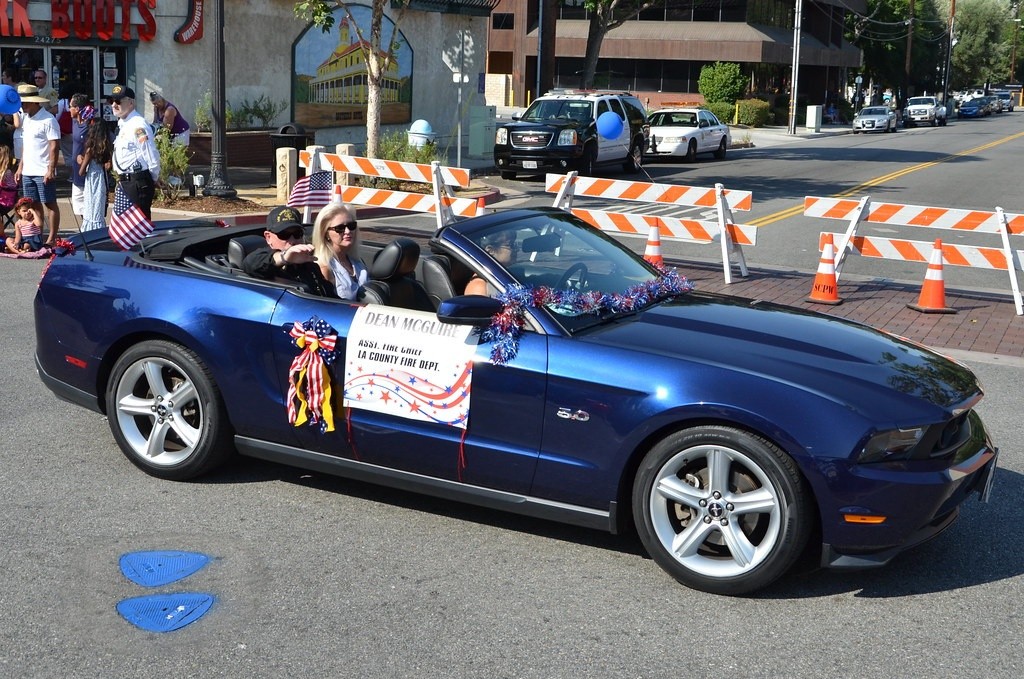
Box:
[280,251,287,265]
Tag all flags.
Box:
[108,186,155,252]
[288,171,333,207]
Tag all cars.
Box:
[642,107,731,163]
[952,89,1014,119]
[853,106,897,135]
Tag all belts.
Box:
[115,172,150,182]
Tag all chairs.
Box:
[359,235,441,308]
[0,185,21,235]
[421,226,494,301]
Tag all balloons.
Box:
[0,84,21,115]
[598,111,623,140]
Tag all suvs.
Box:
[493,90,650,180]
[903,96,942,127]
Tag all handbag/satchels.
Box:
[105,170,116,193]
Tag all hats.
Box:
[17,85,50,102]
[106,85,135,100]
[266,206,303,233]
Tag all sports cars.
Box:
[31,205,1001,599]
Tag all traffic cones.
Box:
[333,185,343,204]
[906,237,958,315]
[803,234,845,306]
[476,197,486,216]
[642,218,662,268]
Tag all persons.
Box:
[103,86,159,223]
[68,95,116,217]
[241,205,325,297]
[5,197,43,255]
[465,230,518,299]
[150,92,189,147]
[0,71,77,239]
[313,202,368,301]
[78,118,111,231]
[822,103,839,122]
[11,85,60,243]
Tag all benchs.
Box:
[226,225,314,268]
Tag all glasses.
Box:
[110,99,121,104]
[328,222,357,233]
[35,76,45,80]
[499,240,517,249]
[68,105,77,108]
[275,229,303,240]
